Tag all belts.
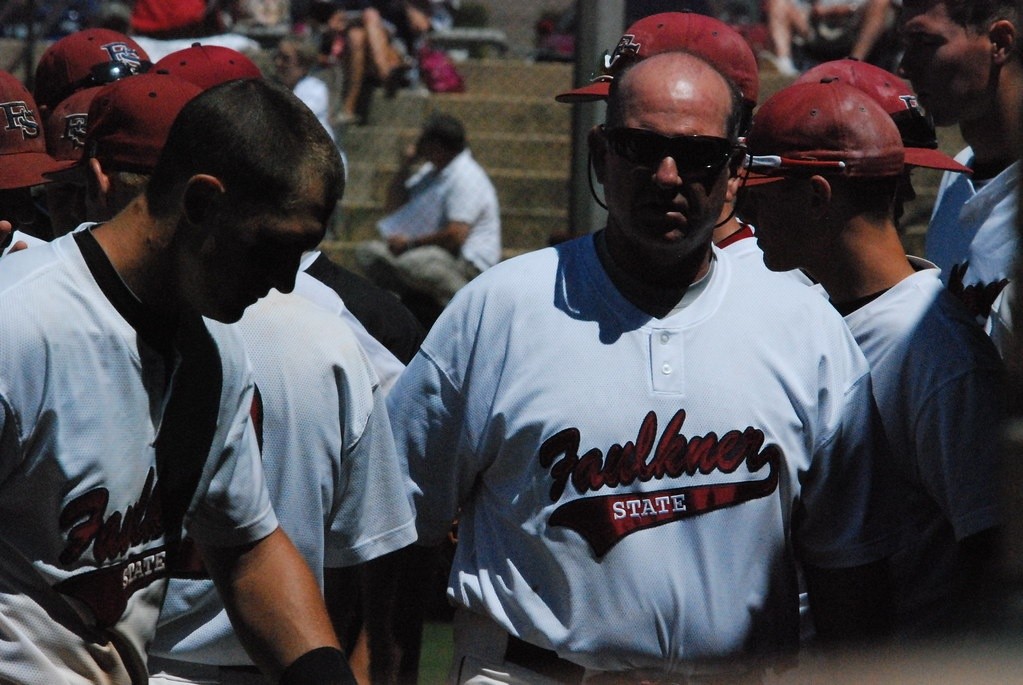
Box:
[507,635,585,685]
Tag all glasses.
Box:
[605,123,734,175]
[42,60,154,101]
[588,48,746,97]
[738,135,845,172]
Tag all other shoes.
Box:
[382,64,421,93]
[756,48,792,76]
[445,46,470,64]
[329,109,364,127]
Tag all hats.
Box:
[32,27,155,111]
[42,85,107,175]
[788,57,976,174]
[554,8,758,104]
[731,76,905,189]
[40,69,203,183]
[148,42,263,91]
[0,70,52,188]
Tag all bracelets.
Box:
[277,646,359,685]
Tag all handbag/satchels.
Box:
[417,47,464,93]
[126,0,208,35]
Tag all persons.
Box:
[41,73,419,685]
[0,78,359,685]
[738,77,1023,639]
[0,0,1023,398]
[368,51,871,685]
